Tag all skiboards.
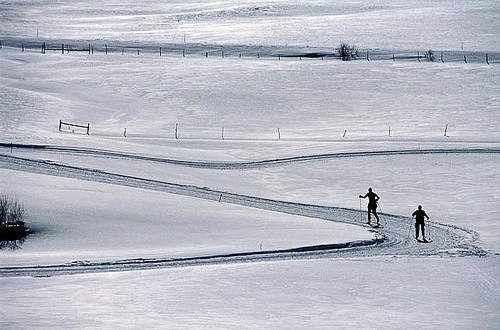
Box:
[413,235,428,243]
[355,220,384,228]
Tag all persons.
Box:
[411,205,430,242]
[359,187,381,226]
